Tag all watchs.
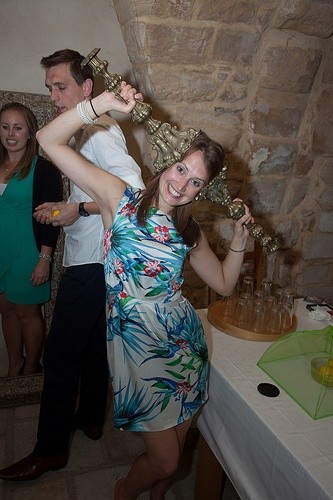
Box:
[79,202,89,217]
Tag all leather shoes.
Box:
[74,408,103,440]
[0,452,68,481]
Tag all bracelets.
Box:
[39,253,51,262]
[77,100,95,125]
[229,247,246,252]
[90,99,99,117]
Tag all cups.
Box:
[220,277,296,333]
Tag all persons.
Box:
[0,102,63,376]
[36,80,255,500]
[0,49,146,482]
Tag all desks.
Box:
[191,296,333,500]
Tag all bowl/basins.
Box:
[310,356,333,387]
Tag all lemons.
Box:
[52,210,59,217]
[319,359,333,376]
